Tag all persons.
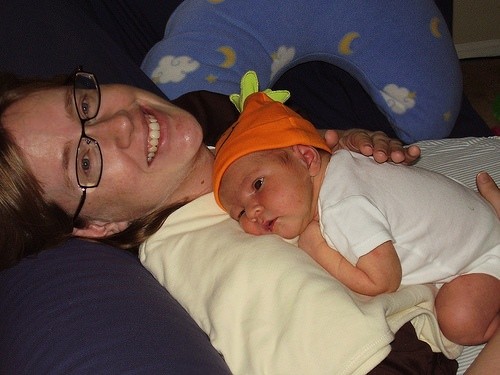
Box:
[216,91,500,347]
[1,74,500,375]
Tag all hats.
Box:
[213,71,332,211]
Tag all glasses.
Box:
[70,68,103,226]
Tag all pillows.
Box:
[142,0,466,143]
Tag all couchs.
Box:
[0,0,493,375]
